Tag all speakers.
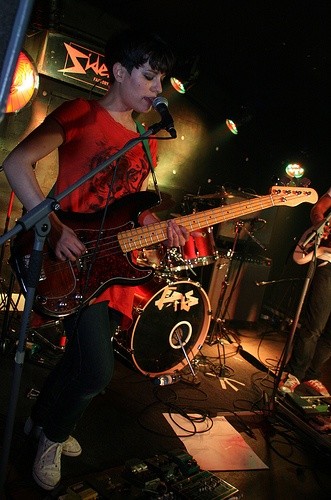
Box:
[201,252,272,324]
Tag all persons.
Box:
[276,187,331,398]
[2,23,190,491]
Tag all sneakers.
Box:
[35,427,82,457]
[278,372,300,394]
[304,379,330,396]
[31,432,66,491]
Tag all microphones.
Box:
[152,97,177,138]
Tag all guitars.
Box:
[10,176,320,318]
[293,223,331,265]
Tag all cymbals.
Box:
[191,190,259,199]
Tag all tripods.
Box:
[207,215,269,343]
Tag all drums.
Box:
[141,226,220,273]
[89,274,212,378]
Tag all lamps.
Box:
[226,101,252,135]
[3,48,40,116]
[169,63,202,94]
[283,149,308,179]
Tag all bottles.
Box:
[156,375,179,385]
